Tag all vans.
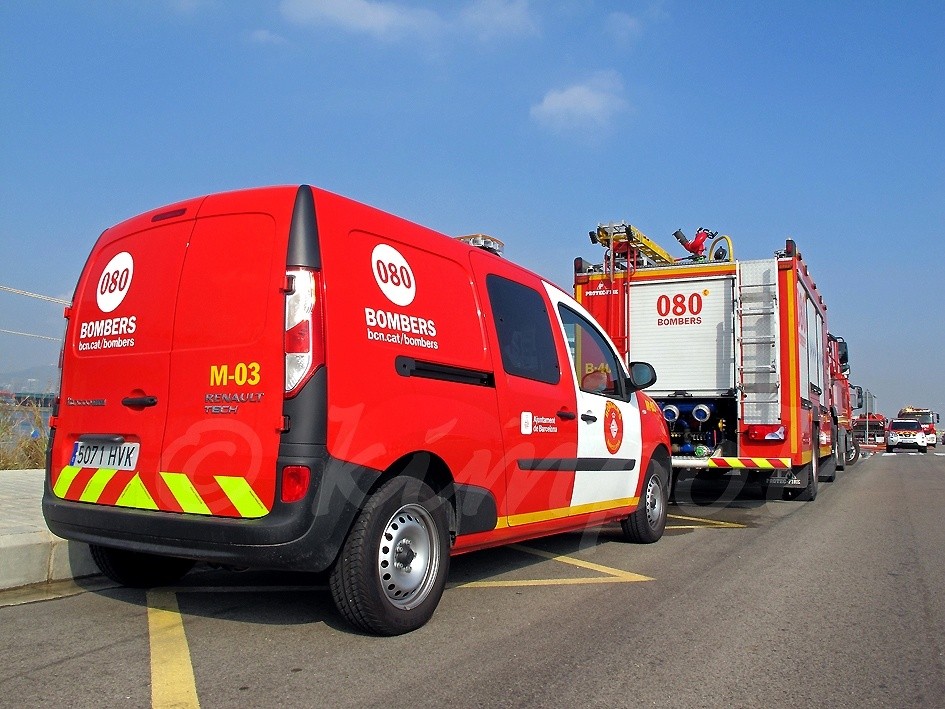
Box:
[40,181,673,637]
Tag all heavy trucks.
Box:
[897,406,940,448]
[575,219,861,502]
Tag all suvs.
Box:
[883,417,928,453]
